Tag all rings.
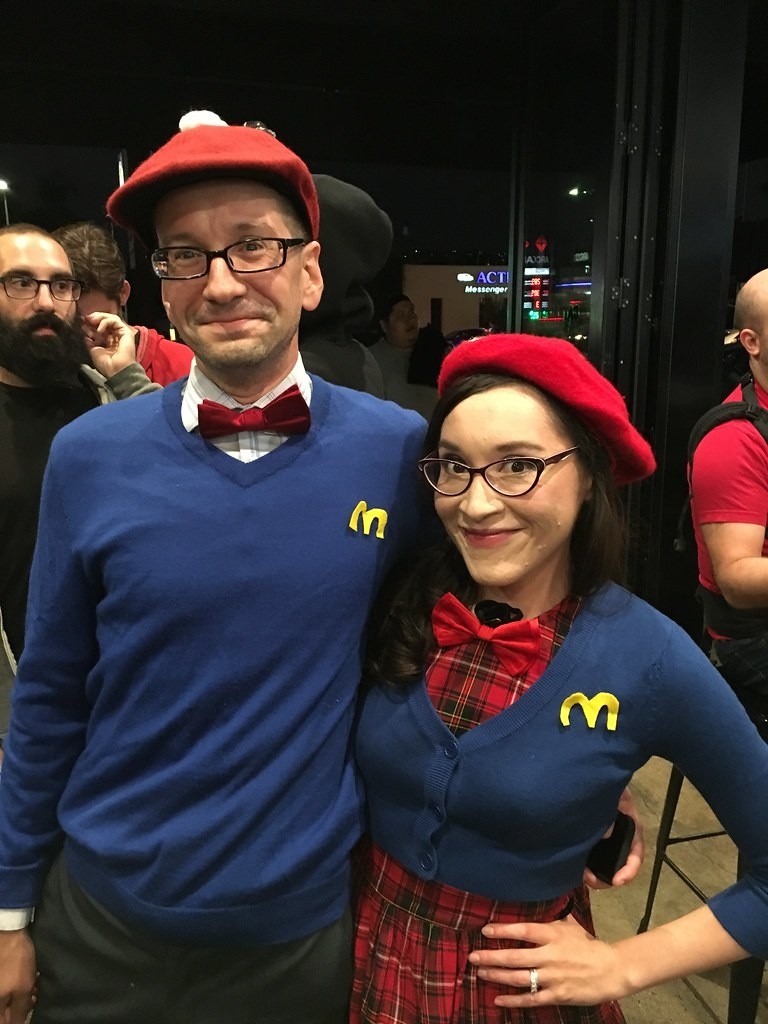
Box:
[529,967,539,995]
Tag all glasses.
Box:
[0,276,86,302]
[416,444,582,498]
[143,238,305,280]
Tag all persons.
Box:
[0,174,504,665]
[687,269,768,741]
[1,110,644,1023]
[347,334,768,1024]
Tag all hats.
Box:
[105,109,322,242]
[437,332,657,486]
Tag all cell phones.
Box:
[586,810,636,885]
